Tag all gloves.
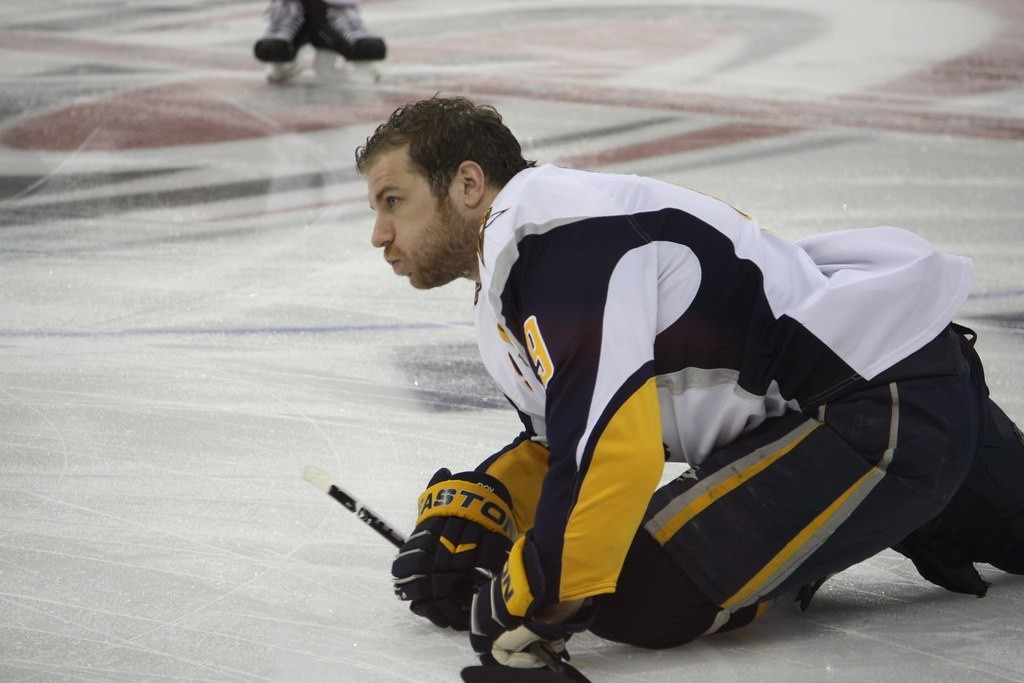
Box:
[460,529,596,683]
[391,467,519,632]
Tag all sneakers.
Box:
[308,0,387,83]
[254,0,318,83]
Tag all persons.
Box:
[363,97,1021,670]
